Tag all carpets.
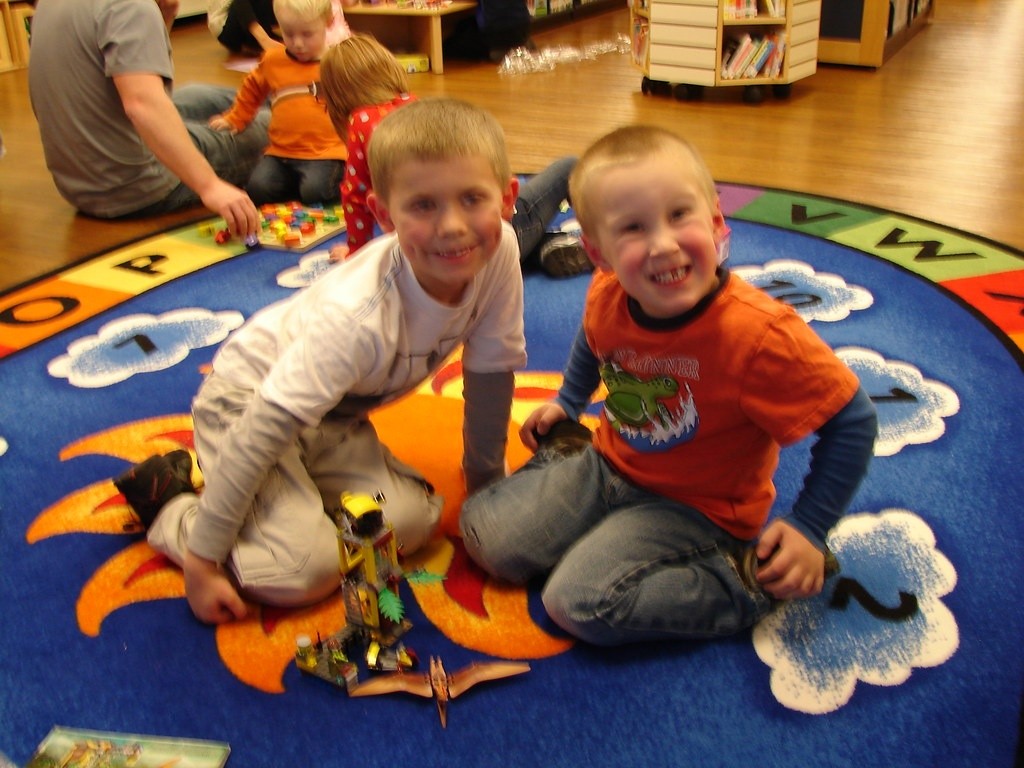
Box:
[3,173,1024,766]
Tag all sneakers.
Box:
[113,450,194,530]
[536,232,595,280]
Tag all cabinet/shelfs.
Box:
[477,0,628,63]
[627,1,819,101]
[822,1,936,68]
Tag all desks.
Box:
[341,0,478,77]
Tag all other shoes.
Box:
[241,45,264,57]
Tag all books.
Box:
[720,0,788,83]
[524,1,549,20]
[631,0,649,71]
[548,0,574,15]
[580,0,599,5]
[21,722,231,768]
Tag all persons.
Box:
[205,0,352,209]
[111,102,528,620]
[315,32,595,282]
[25,0,271,246]
[205,1,287,56]
[455,124,878,654]
